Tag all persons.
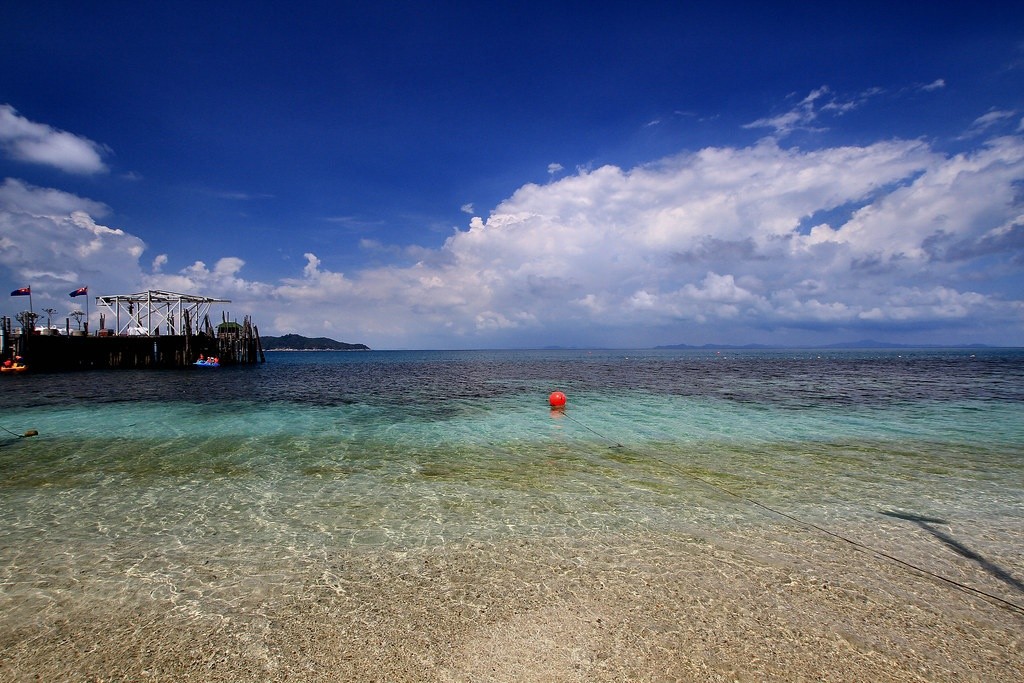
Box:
[199,354,219,363]
[3,358,13,368]
[15,356,23,366]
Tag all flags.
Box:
[69,287,87,297]
[10,288,30,296]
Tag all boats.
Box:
[1,362,28,372]
[194,359,221,368]
